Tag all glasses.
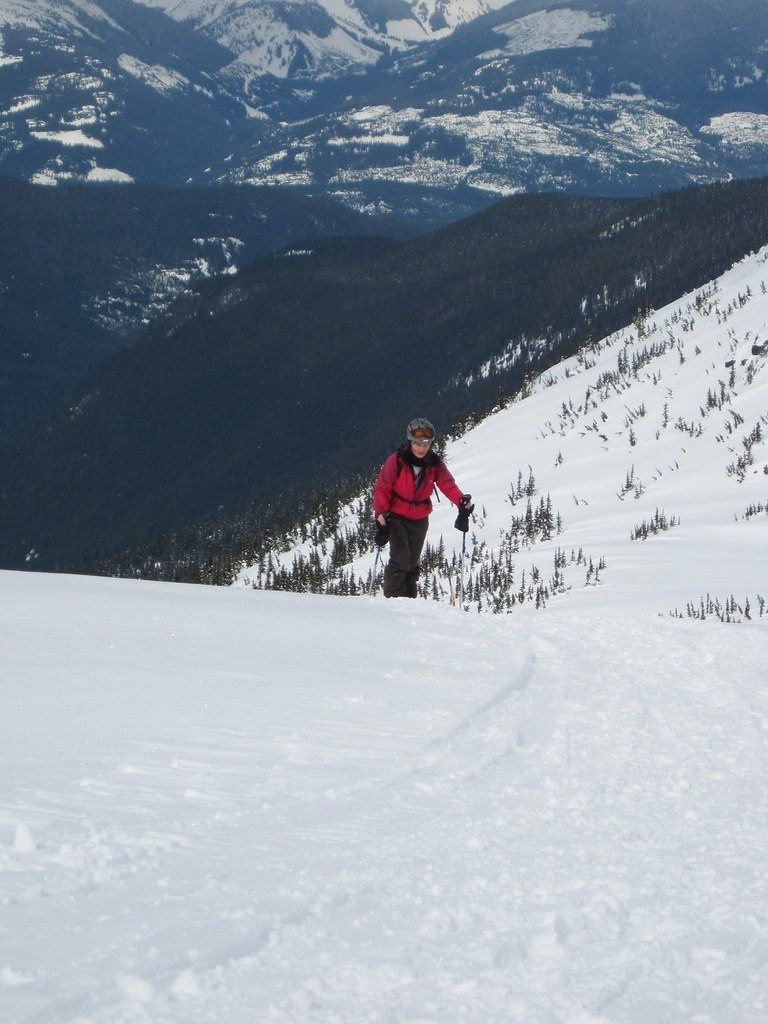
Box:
[412,428,434,439]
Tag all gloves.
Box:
[374,511,392,546]
[455,494,474,532]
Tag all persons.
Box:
[373,419,471,599]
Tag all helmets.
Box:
[407,418,435,443]
